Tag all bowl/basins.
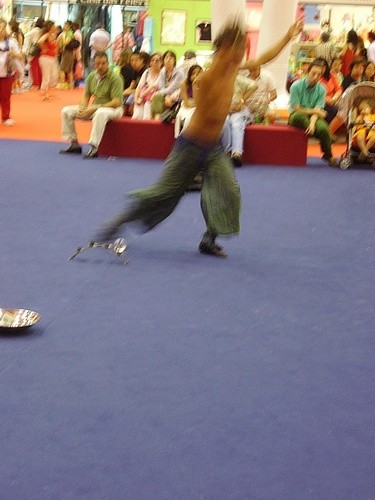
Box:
[0,308,41,333]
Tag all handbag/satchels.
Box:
[159,100,182,125]
[5,37,26,78]
[29,45,40,58]
[75,61,84,80]
[65,39,80,52]
[138,84,160,100]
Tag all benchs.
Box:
[100,116,309,166]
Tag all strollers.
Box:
[339,80,375,170]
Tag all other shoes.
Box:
[2,120,14,126]
[197,240,227,256]
[230,153,242,167]
[82,150,97,159]
[321,155,339,166]
[58,145,82,155]
[55,81,74,91]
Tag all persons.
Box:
[94,8,305,259]
[0,16,375,171]
